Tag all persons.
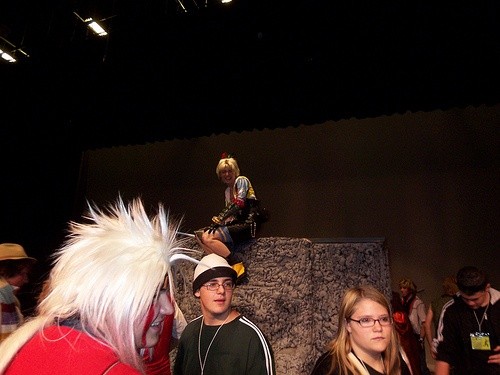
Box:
[485,340,488,347]
[435,268,500,375]
[195,152,263,282]
[171,253,275,375]
[0,193,216,375]
[0,243,35,339]
[425,278,458,359]
[310,284,421,375]
[399,279,430,375]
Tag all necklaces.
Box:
[198,311,233,375]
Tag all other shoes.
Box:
[227,255,248,283]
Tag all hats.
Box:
[0,243,36,266]
[192,253,237,299]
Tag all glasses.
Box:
[348,315,395,326]
[202,283,236,291]
